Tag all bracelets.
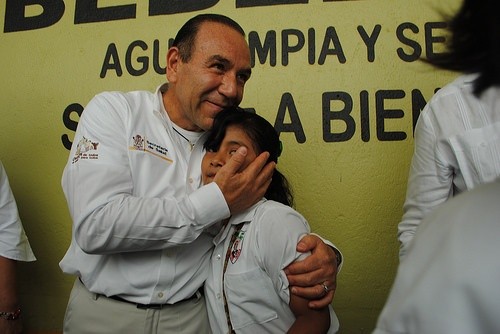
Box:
[0,303,24,320]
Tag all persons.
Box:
[201,106,340,334]
[396,0,500,264]
[59,13,344,334]
[371,172,500,334]
[0,159,36,334]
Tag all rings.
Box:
[321,283,330,292]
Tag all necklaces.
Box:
[172,124,195,151]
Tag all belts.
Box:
[78,277,204,309]
